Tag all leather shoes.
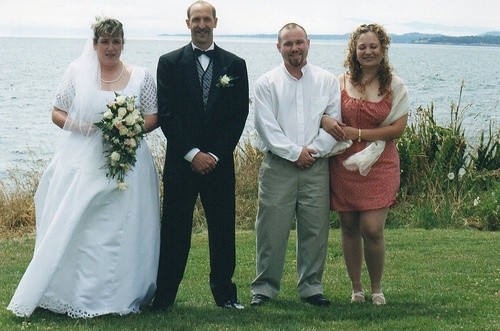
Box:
[251,294,268,306]
[223,301,244,310]
[140,294,170,312]
[304,294,330,304]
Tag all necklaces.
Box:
[100,61,124,83]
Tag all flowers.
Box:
[216,74,233,88]
[93,90,147,193]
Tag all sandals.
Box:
[351,287,365,302]
[371,292,386,305]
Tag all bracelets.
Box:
[358,128,361,141]
[320,114,328,127]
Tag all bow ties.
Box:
[194,48,214,58]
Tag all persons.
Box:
[51,18,161,318]
[321,24,409,303]
[251,23,342,307]
[152,0,249,311]
